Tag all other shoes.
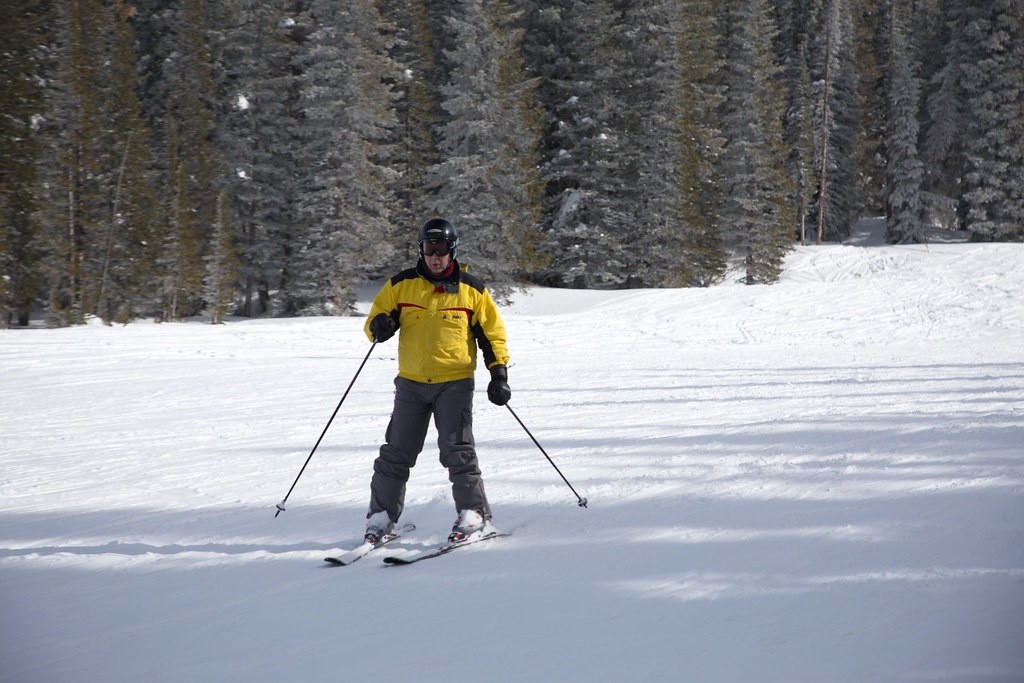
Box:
[447,508,493,544]
[364,511,393,545]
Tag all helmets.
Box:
[417,219,457,259]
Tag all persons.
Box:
[363,217,512,542]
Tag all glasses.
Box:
[419,239,453,257]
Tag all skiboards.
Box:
[323,521,512,565]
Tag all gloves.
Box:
[487,364,511,405]
[370,313,395,343]
